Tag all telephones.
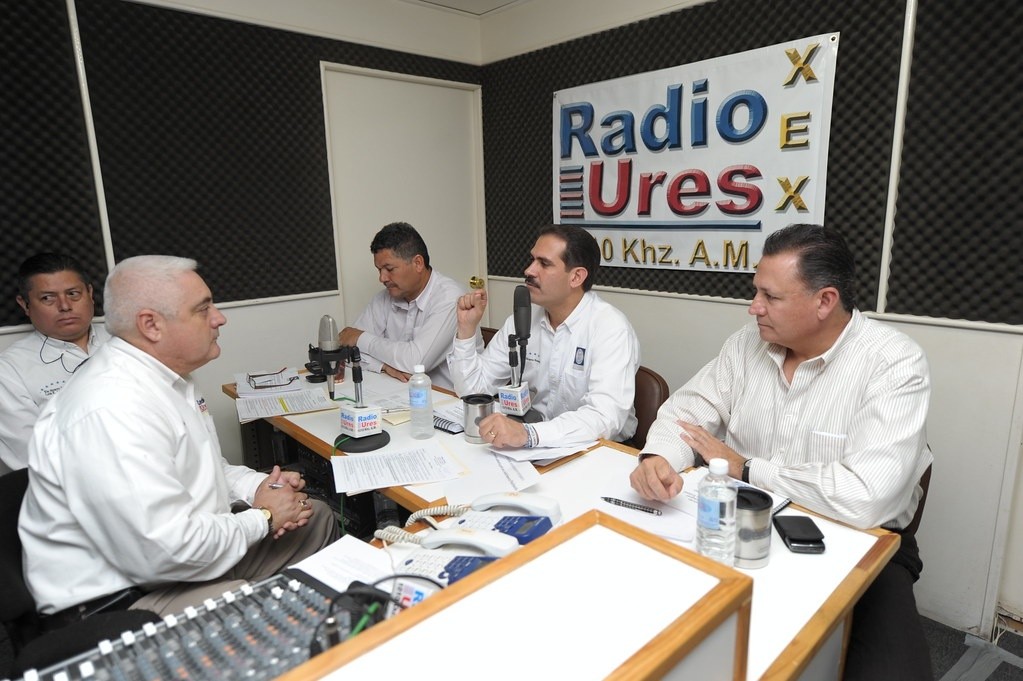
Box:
[452,493,563,546]
[392,528,520,591]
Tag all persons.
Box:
[445,224,642,451]
[18,255,340,619]
[629,224,935,681]
[0,252,113,483]
[339,222,485,390]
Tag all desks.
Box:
[221,361,600,515]
[371,439,902,681]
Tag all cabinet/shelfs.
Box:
[267,508,753,681]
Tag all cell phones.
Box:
[773,515,825,554]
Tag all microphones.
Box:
[514,285,532,374]
[319,315,339,399]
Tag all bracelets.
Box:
[522,423,537,448]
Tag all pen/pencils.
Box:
[601,495,663,516]
[269,483,283,488]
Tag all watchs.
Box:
[742,459,752,483]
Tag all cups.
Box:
[463,393,496,444]
[733,487,775,567]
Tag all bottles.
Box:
[699,462,737,571]
[408,364,435,439]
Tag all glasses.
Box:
[246,367,295,389]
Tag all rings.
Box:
[299,500,309,510]
[487,431,496,439]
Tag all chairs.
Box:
[631,365,669,451]
[481,327,499,349]
[0,466,147,630]
